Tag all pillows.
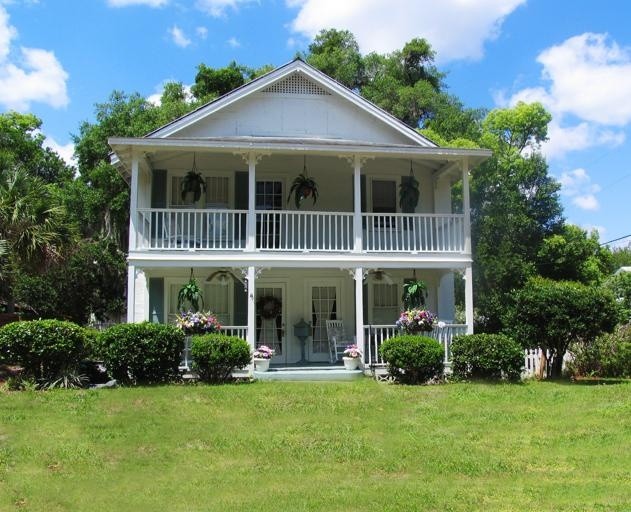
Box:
[327,327,346,344]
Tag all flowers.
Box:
[344,344,363,360]
[395,307,446,332]
[175,304,221,336]
[254,345,274,359]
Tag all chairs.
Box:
[160,215,202,247]
[326,319,347,363]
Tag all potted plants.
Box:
[176,280,204,313]
[286,174,319,209]
[179,171,207,202]
[398,280,429,306]
[398,176,420,210]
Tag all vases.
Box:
[409,327,427,332]
[254,359,270,371]
[341,356,358,370]
